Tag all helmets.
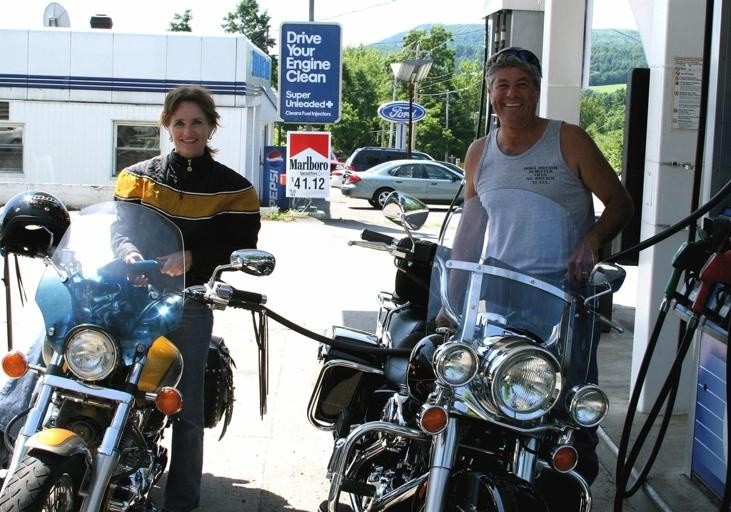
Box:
[0,188,70,259]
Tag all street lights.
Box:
[391,58,433,161]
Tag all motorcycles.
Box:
[1,193,276,511]
[306,192,629,512]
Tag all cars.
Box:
[341,158,467,211]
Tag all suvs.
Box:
[345,146,437,172]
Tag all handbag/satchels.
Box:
[205,336,234,426]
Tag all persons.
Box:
[435,45,636,512]
[108,86,261,512]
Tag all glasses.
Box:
[496,49,542,78]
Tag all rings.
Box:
[170,272,175,277]
